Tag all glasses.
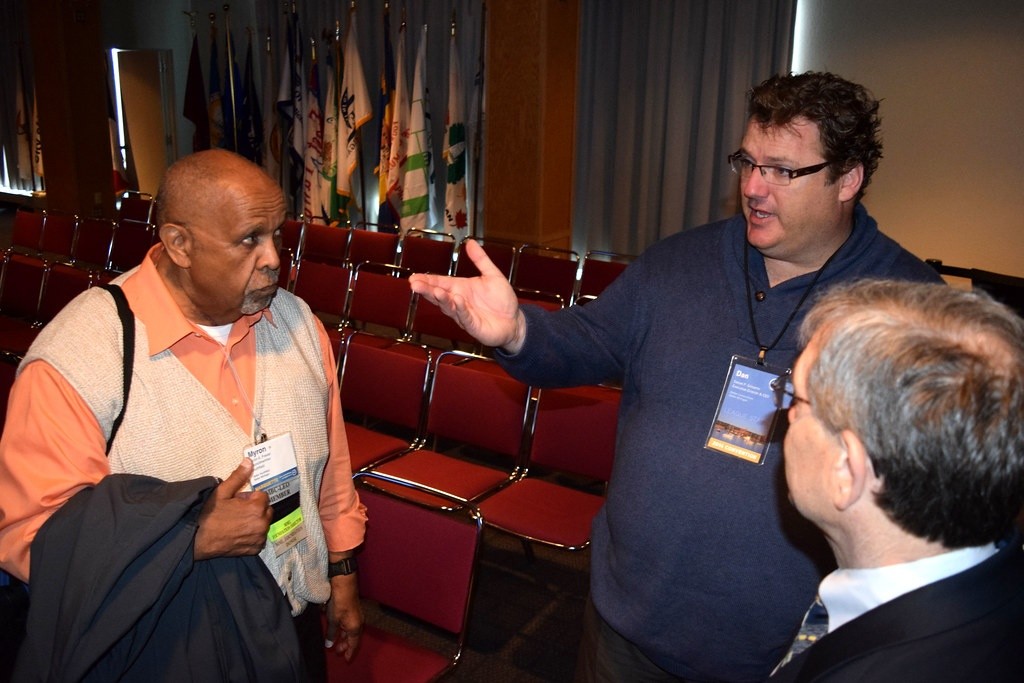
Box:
[728,149,847,186]
[771,369,882,479]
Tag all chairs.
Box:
[1,190,636,682]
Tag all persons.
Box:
[770,281,1024,683]
[409,69,949,683]
[0,148,368,683]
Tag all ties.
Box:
[769,594,829,677]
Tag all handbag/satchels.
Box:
[0,581,30,683]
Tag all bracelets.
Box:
[327,556,358,577]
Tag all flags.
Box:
[15,56,43,181]
[183,10,468,242]
